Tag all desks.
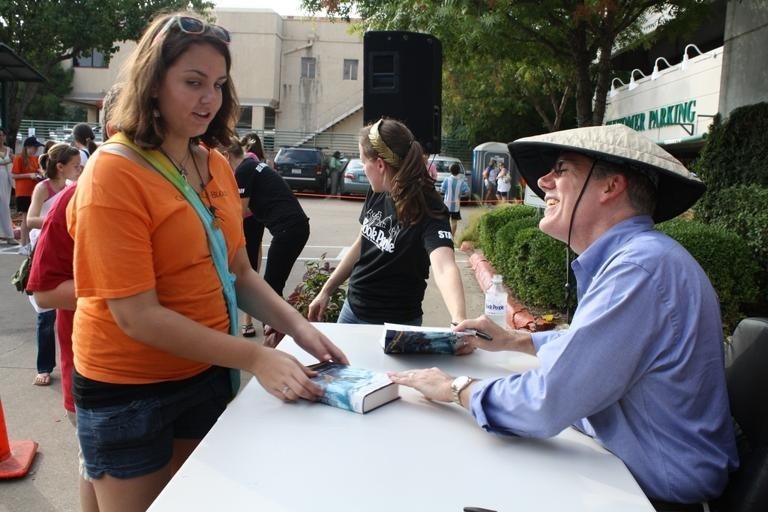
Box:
[145,321,656,511]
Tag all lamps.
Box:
[681,44,716,71]
[628,69,651,91]
[650,57,679,81]
[609,77,629,98]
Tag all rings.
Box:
[281,386,290,394]
[407,372,415,378]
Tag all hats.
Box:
[24,137,44,147]
[507,123,707,224]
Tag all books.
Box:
[301,360,403,414]
[380,322,476,354]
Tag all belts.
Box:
[647,494,714,510]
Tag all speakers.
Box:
[363,30,443,155]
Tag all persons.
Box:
[494,162,512,204]
[0,114,311,385]
[304,117,468,330]
[481,158,500,208]
[64,11,351,512]
[439,163,470,241]
[384,121,745,512]
[421,149,437,185]
[23,80,127,512]
[328,150,342,197]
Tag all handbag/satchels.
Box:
[10,258,33,295]
[221,273,239,396]
[503,172,512,183]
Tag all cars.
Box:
[340,160,381,195]
[429,157,469,200]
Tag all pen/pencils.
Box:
[450,322,493,342]
[464,507,497,512]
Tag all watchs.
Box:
[450,372,482,408]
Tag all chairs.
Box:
[684,317,768,511]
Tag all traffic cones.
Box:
[1,406,37,477]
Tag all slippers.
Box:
[242,323,255,336]
[35,374,50,386]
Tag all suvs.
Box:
[273,144,327,191]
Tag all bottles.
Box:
[485,274,507,331]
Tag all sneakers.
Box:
[7,238,19,245]
[18,244,31,256]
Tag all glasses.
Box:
[553,161,605,173]
[151,14,230,46]
